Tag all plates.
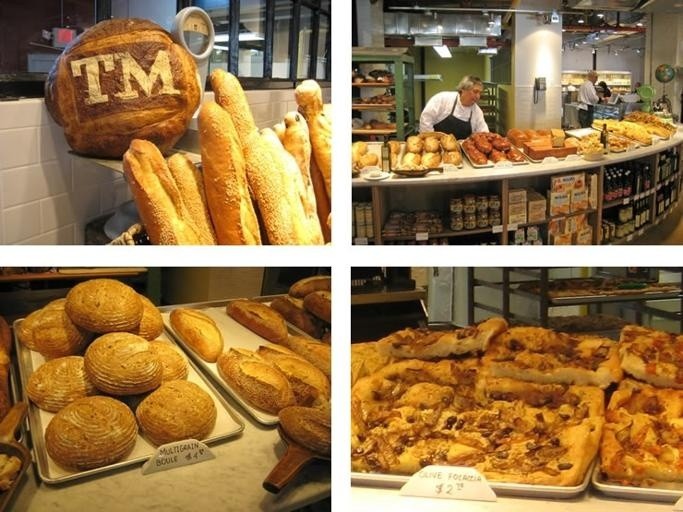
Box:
[363,172,388,182]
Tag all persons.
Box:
[415,74,491,140]
[597,81,612,105]
[576,70,600,130]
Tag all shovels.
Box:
[262,424,330,493]
[1,402,33,511]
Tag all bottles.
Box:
[601,149,679,242]
[600,123,610,154]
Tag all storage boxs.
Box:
[508,171,598,244]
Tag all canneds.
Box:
[448,193,500,231]
[352,201,374,238]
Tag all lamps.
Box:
[395,6,648,59]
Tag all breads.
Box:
[43,18,332,246]
[518,275,682,300]
[352,110,679,174]
[1,275,331,490]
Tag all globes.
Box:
[656,64,675,113]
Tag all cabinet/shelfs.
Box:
[466,265,681,343]
[658,140,681,219]
[374,180,505,244]
[352,51,413,143]
[471,83,497,127]
[351,181,378,244]
[505,166,602,243]
[603,147,657,246]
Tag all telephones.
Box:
[536,78,546,92]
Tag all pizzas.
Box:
[351,315,683,490]
